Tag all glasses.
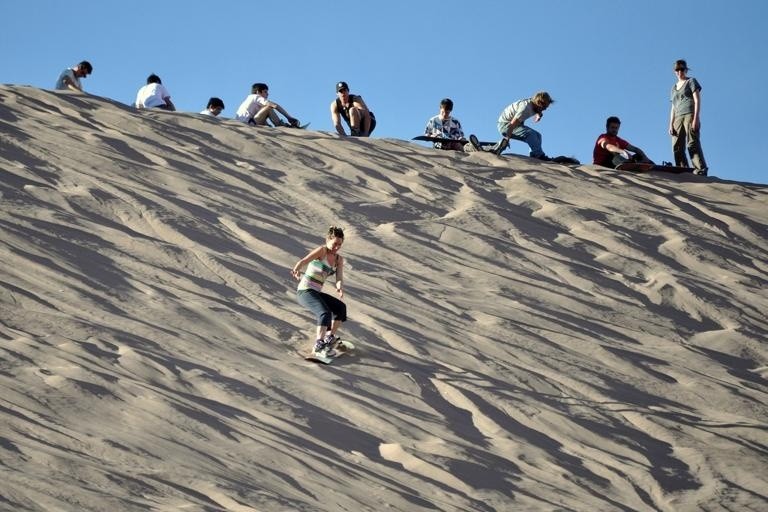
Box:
[81,71,86,78]
[675,67,685,72]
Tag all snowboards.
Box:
[502,152,555,163]
[412,136,498,145]
[615,163,695,174]
[306,341,355,364]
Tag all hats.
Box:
[336,82,348,91]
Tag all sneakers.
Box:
[275,119,288,127]
[326,334,341,349]
[530,152,551,161]
[493,139,507,156]
[313,340,336,356]
[693,168,708,175]
[470,135,483,152]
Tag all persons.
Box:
[52,60,92,93]
[134,72,176,110]
[424,97,508,156]
[196,96,225,118]
[234,82,309,129]
[496,89,556,161]
[667,58,707,176]
[329,80,375,137]
[591,115,655,171]
[289,225,347,360]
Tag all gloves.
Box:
[289,118,300,127]
